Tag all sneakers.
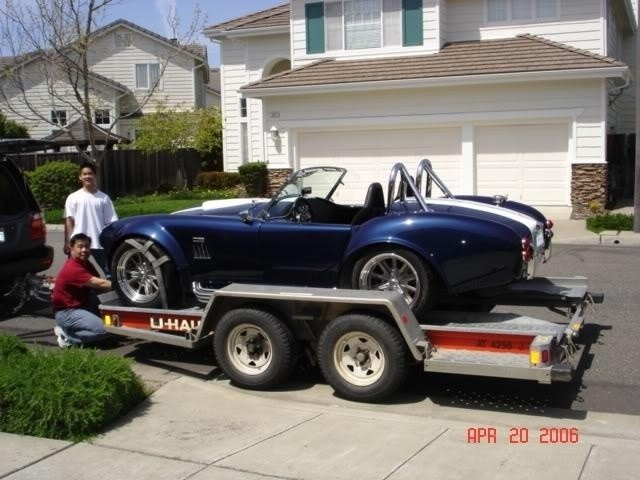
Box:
[53,324,71,349]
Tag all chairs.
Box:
[331,176,414,225]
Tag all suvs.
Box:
[0,136,70,324]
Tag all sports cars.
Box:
[96,153,556,320]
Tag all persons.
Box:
[50,234,122,351]
[60,161,120,315]
